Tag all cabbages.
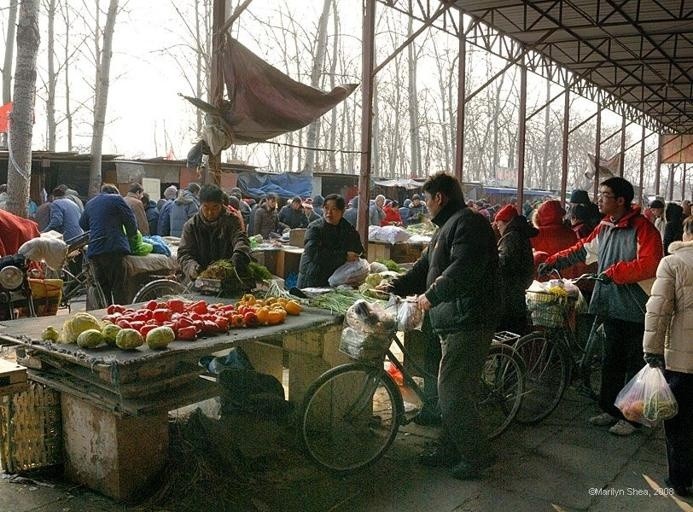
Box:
[42,312,176,350]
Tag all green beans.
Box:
[643,389,678,423]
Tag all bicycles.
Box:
[56,231,110,317]
[498,263,611,427]
[129,262,200,310]
[295,286,531,466]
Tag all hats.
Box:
[494,203,518,223]
[648,200,664,210]
[53,184,69,197]
[570,190,591,205]
[536,199,567,226]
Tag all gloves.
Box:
[227,249,251,278]
[184,261,203,281]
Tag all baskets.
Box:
[0,378,64,474]
[337,314,393,363]
[523,290,578,330]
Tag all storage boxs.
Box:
[289,228,307,247]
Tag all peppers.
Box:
[100,292,302,340]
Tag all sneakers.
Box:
[448,439,497,481]
[665,475,693,497]
[608,419,642,436]
[410,437,461,466]
[589,411,617,427]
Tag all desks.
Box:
[366,238,431,265]
[112,238,306,303]
[0,292,388,504]
